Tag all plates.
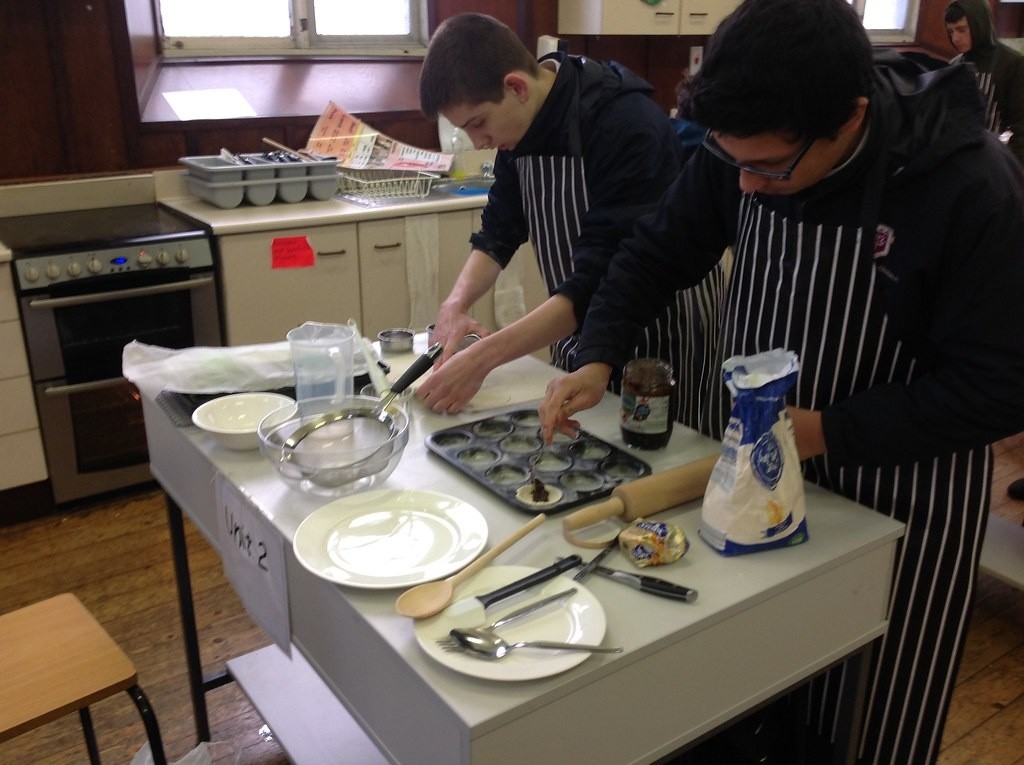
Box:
[412,563,607,680]
[292,488,489,590]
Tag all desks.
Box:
[142,328,909,765]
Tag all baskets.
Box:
[338,168,442,205]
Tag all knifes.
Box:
[573,530,620,583]
[555,554,699,604]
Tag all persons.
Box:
[414,13,723,432]
[538,0,1024,765]
[944,0,1024,153]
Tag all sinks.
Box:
[428,176,496,197]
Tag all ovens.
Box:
[15,273,229,507]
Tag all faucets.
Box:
[481,161,495,179]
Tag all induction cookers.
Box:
[0,200,222,295]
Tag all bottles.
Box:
[620,358,678,450]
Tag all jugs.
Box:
[287,322,357,405]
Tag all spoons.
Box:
[449,627,624,661]
[395,513,546,619]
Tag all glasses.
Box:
[702,126,818,183]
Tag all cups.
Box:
[426,324,435,349]
[360,379,416,424]
[454,333,482,354]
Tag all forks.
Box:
[434,587,579,655]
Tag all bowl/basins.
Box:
[377,327,415,352]
[191,392,298,449]
[254,397,410,502]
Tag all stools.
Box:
[0,591,167,765]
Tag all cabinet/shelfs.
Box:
[215,207,554,366]
[0,260,47,491]
[558,0,742,35]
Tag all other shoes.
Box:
[1007,477,1024,502]
